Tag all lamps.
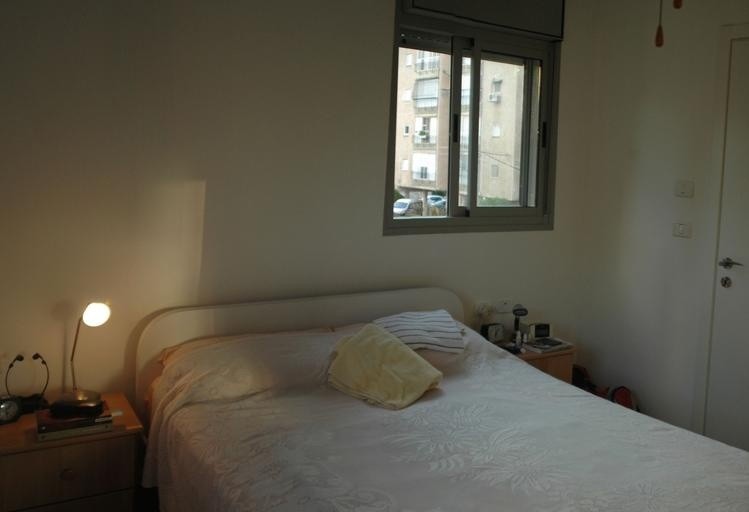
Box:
[62,299,112,403]
[513,307,529,343]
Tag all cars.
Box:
[426,195,445,208]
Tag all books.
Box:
[33,422,114,441]
[35,406,114,433]
[523,341,572,353]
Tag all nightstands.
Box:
[495,332,577,385]
[0,392,145,512]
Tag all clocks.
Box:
[0,394,21,424]
[482,322,503,344]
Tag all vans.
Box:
[392,198,422,217]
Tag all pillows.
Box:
[160,327,335,402]
[333,325,469,377]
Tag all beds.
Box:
[134,288,749,512]
